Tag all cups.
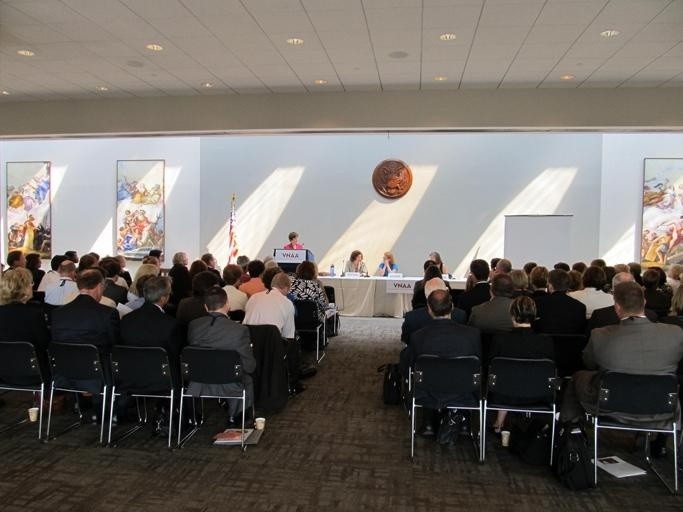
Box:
[255,417,266,429]
[501,431,510,447]
[28,408,38,422]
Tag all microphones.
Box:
[302,242,304,248]
[340,260,344,275]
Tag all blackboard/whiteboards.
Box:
[504,214,574,270]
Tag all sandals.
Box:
[490,424,504,437]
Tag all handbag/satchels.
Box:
[554,421,593,491]
[377,362,403,405]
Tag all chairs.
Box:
[407,354,482,465]
[176,347,255,452]
[227,309,245,321]
[584,370,679,497]
[534,334,588,362]
[482,356,557,467]
[241,324,296,400]
[43,339,143,445]
[293,300,326,365]
[324,285,335,303]
[106,345,197,449]
[0,342,82,441]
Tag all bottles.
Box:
[330,265,334,276]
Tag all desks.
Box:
[317,276,468,317]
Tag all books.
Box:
[590,454,649,479]
[215,428,256,444]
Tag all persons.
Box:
[379,252,399,276]
[0,249,330,425]
[118,176,164,250]
[642,176,683,265]
[7,175,50,255]
[402,252,683,469]
[284,231,303,250]
[344,249,368,277]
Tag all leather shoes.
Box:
[150,405,180,439]
[416,421,434,440]
[226,413,241,428]
